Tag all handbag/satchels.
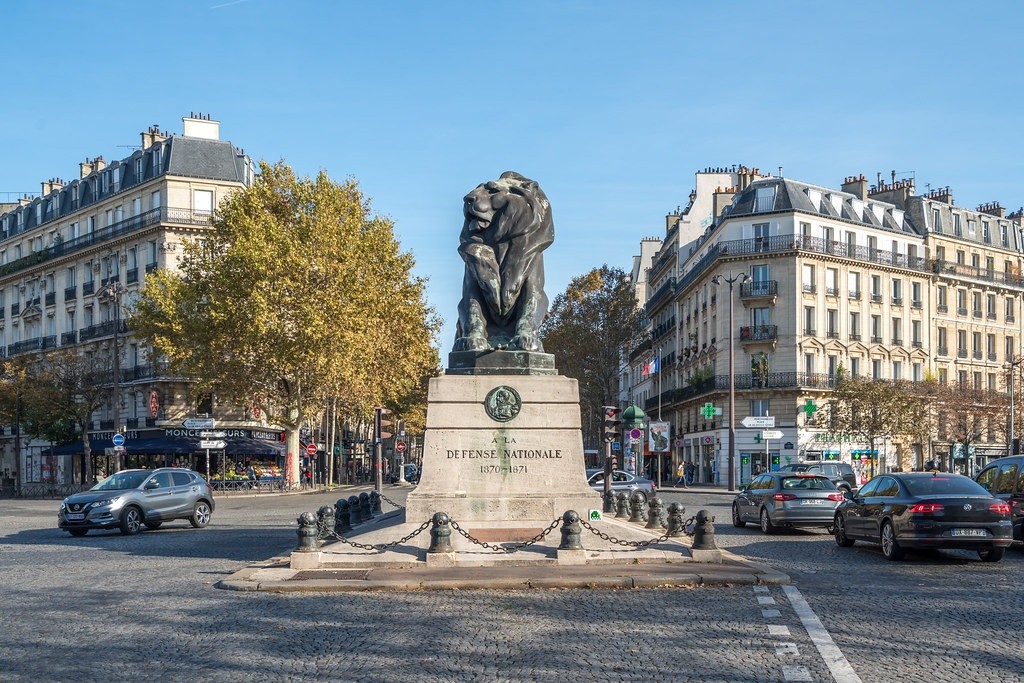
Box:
[677,465,683,477]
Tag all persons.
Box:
[299,463,311,483]
[236,460,258,489]
[924,460,940,473]
[365,464,369,482]
[673,460,689,489]
[416,464,422,485]
[245,456,251,468]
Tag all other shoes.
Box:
[673,485,676,489]
[685,486,688,489]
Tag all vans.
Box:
[970,454,1024,552]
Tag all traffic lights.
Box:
[601,406,621,443]
[373,407,391,443]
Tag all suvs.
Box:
[775,460,857,497]
[58,467,216,536]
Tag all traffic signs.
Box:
[763,431,785,440]
[740,416,775,428]
[181,417,215,430]
[200,440,229,449]
[200,431,227,438]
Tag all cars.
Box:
[732,471,846,535]
[833,470,1013,562]
[586,468,657,504]
[391,463,419,485]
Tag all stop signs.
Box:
[396,442,408,452]
[307,444,317,455]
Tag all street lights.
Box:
[710,270,754,491]
[1002,353,1024,455]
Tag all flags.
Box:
[641,354,658,374]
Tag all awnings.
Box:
[219,437,278,456]
[126,437,198,454]
[255,437,308,456]
[42,438,115,456]
